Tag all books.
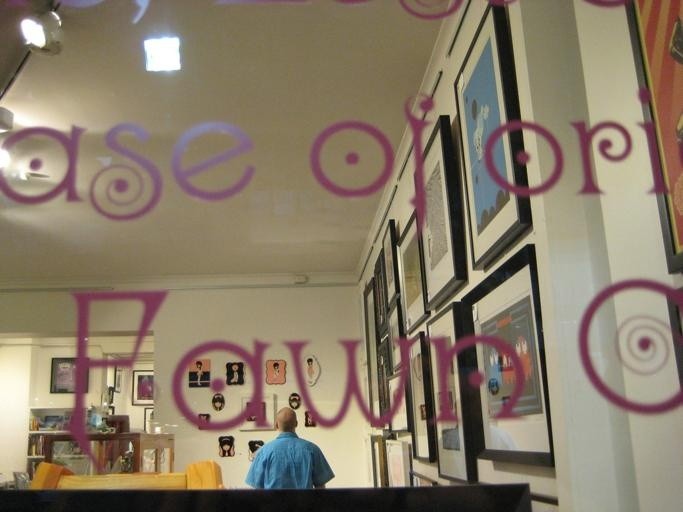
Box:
[26,433,45,456]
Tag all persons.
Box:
[289,393,301,409]
[306,358,315,380]
[195,361,203,386]
[218,436,236,457]
[244,407,335,491]
[271,362,281,380]
[212,393,224,411]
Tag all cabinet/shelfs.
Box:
[27,405,93,480]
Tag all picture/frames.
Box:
[49,357,88,393]
[105,355,154,432]
[361,5,556,487]
[623,1,682,272]
[238,393,275,432]
